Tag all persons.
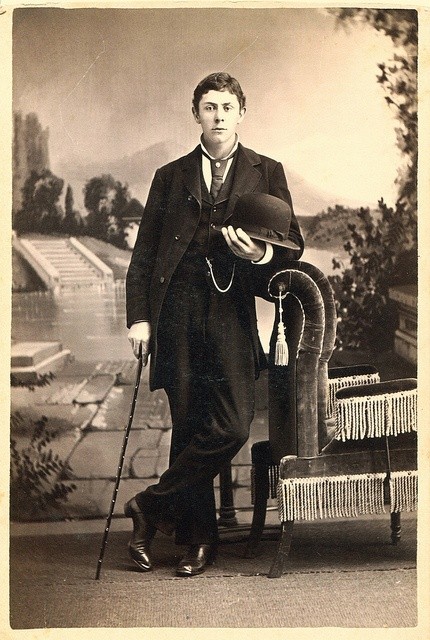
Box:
[125,69,305,575]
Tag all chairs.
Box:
[236,261,417,579]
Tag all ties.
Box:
[201,150,238,201]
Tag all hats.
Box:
[212,192,301,251]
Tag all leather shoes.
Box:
[176,544,215,576]
[124,497,157,571]
